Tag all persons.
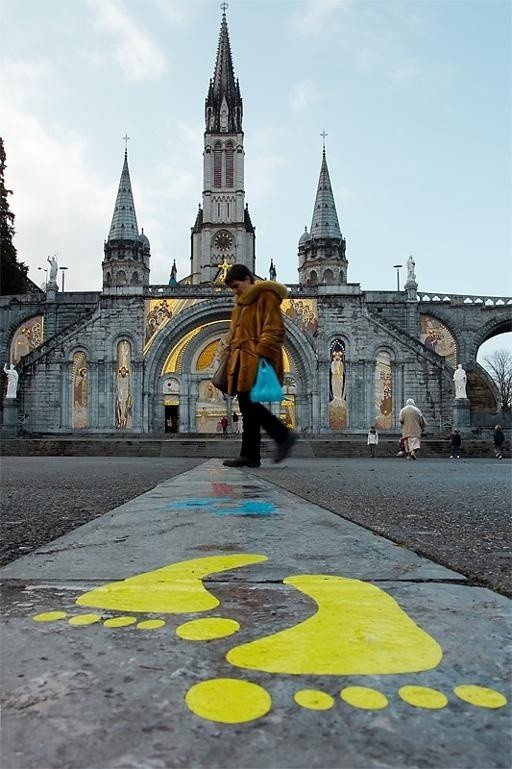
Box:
[116,366,130,422]
[219,264,302,470]
[366,426,379,458]
[448,434,454,446]
[74,368,88,408]
[220,414,230,435]
[231,411,240,434]
[145,299,173,339]
[397,399,428,458]
[330,351,344,400]
[452,364,468,398]
[449,431,461,459]
[492,426,504,459]
[14,327,35,363]
[3,363,20,398]
[48,256,59,282]
[286,299,319,337]
[405,255,416,280]
[422,329,446,353]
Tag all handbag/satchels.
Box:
[250,357,283,401]
[211,349,240,396]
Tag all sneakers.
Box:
[223,456,261,467]
[272,431,300,463]
[407,452,417,460]
